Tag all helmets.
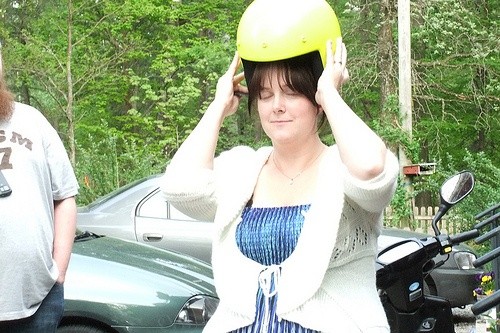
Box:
[236,0,342,74]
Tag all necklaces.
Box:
[273,147,324,185]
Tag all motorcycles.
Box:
[376,170,480,333]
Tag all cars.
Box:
[58,228,220,333]
[76,173,485,309]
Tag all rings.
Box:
[335,61,342,64]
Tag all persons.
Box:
[162,0,399,333]
[0,51,80,333]
